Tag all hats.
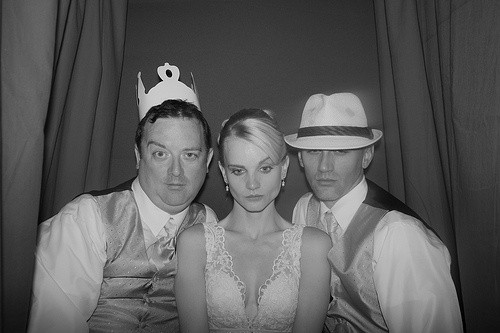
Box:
[283,92,384,149]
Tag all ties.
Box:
[146,220,177,271]
[325,212,341,246]
